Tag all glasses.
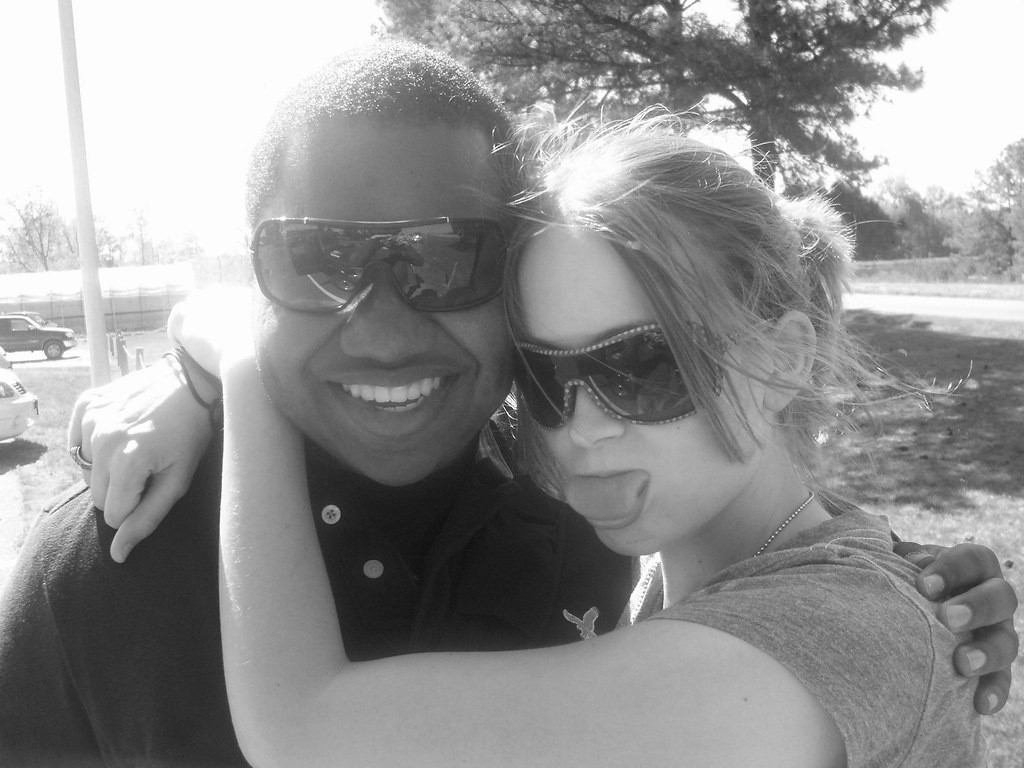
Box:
[514,318,778,431]
[247,217,508,313]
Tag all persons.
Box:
[169,109,988,768]
[0,41,1019,768]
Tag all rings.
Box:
[69,446,93,471]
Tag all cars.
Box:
[0,368,40,442]
[8,311,61,330]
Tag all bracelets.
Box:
[162,345,226,433]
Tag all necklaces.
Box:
[753,487,816,558]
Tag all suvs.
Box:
[0,315,78,360]
